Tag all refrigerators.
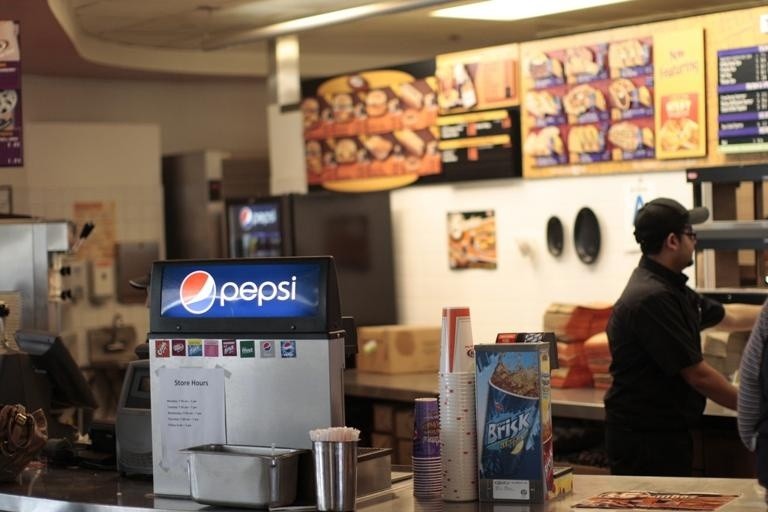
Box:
[222,190,396,326]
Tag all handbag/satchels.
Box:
[0,404,49,481]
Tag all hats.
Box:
[633,198,710,243]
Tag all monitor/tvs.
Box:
[13,333,100,438]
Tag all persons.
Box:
[603,197,764,477]
[738,298,766,490]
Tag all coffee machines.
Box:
[0,210,95,355]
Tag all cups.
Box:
[311,439,358,510]
[481,381,540,477]
[410,395,442,497]
[439,304,480,503]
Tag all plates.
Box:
[545,215,564,257]
[573,207,601,266]
